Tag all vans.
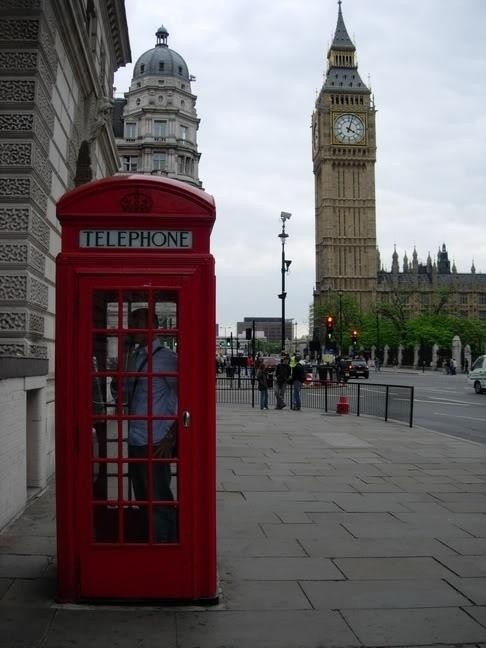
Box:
[467,355,486,393]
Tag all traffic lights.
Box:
[352,331,357,344]
[237,342,240,348]
[227,338,231,347]
[327,317,334,332]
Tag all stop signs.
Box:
[302,372,313,385]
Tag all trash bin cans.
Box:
[226,368,235,377]
[317,365,329,380]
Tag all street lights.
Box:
[220,326,232,355]
[337,288,345,357]
[279,211,292,354]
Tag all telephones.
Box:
[125,335,133,353]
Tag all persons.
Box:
[292,355,305,410]
[442,358,469,376]
[109,307,178,544]
[273,356,291,409]
[256,363,269,410]
[216,352,296,385]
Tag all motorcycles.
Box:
[335,357,352,382]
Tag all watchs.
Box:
[166,431,176,440]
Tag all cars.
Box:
[258,357,280,372]
[349,360,369,379]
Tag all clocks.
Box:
[333,113,366,145]
